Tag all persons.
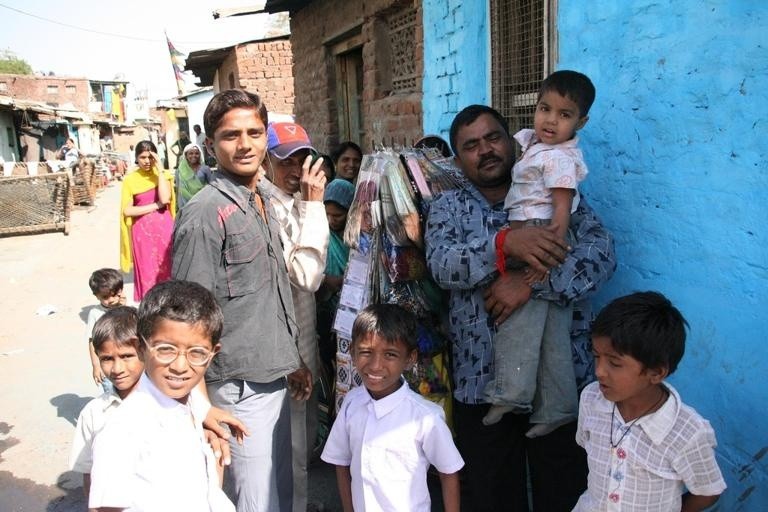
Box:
[168,71,620,512]
[119,125,218,303]
[66,261,250,493]
[86,280,241,511]
[569,292,727,512]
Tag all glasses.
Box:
[139,331,216,366]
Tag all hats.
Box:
[266,122,319,161]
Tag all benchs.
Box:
[0,156,122,238]
